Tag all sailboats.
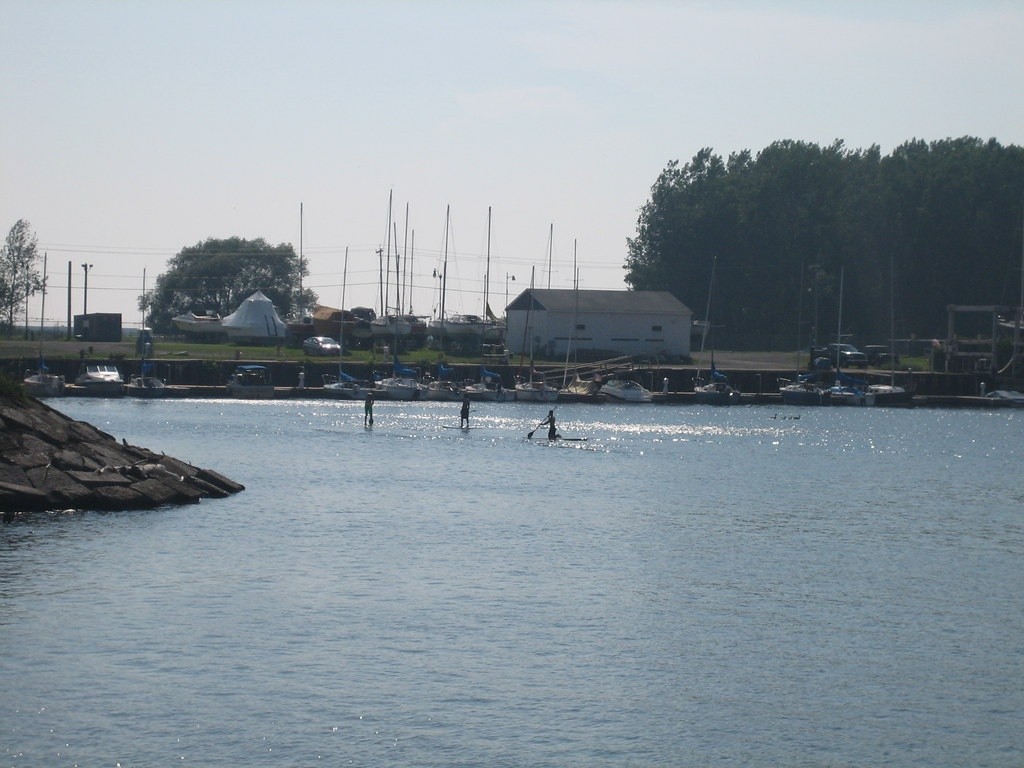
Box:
[465,274,516,402]
[865,256,916,408]
[287,189,507,343]
[515,266,559,402]
[694,317,741,404]
[122,267,170,398]
[375,255,420,400]
[417,274,466,402]
[322,246,377,399]
[558,239,605,404]
[24,253,65,396]
[830,267,866,407]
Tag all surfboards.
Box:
[522,437,588,441]
[443,426,483,430]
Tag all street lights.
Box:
[506,272,516,306]
[433,269,442,319]
[81,262,93,341]
[433,269,442,318]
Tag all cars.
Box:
[828,344,869,369]
[863,345,900,369]
[303,337,342,356]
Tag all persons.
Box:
[460,395,470,428]
[364,391,375,425]
[542,410,556,438]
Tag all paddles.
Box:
[528,406,558,437]
[458,410,477,418]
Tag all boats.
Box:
[226,365,274,398]
[600,379,653,402]
[482,344,507,363]
[75,363,125,396]
[779,379,824,403]
[172,310,223,333]
[222,290,287,345]
